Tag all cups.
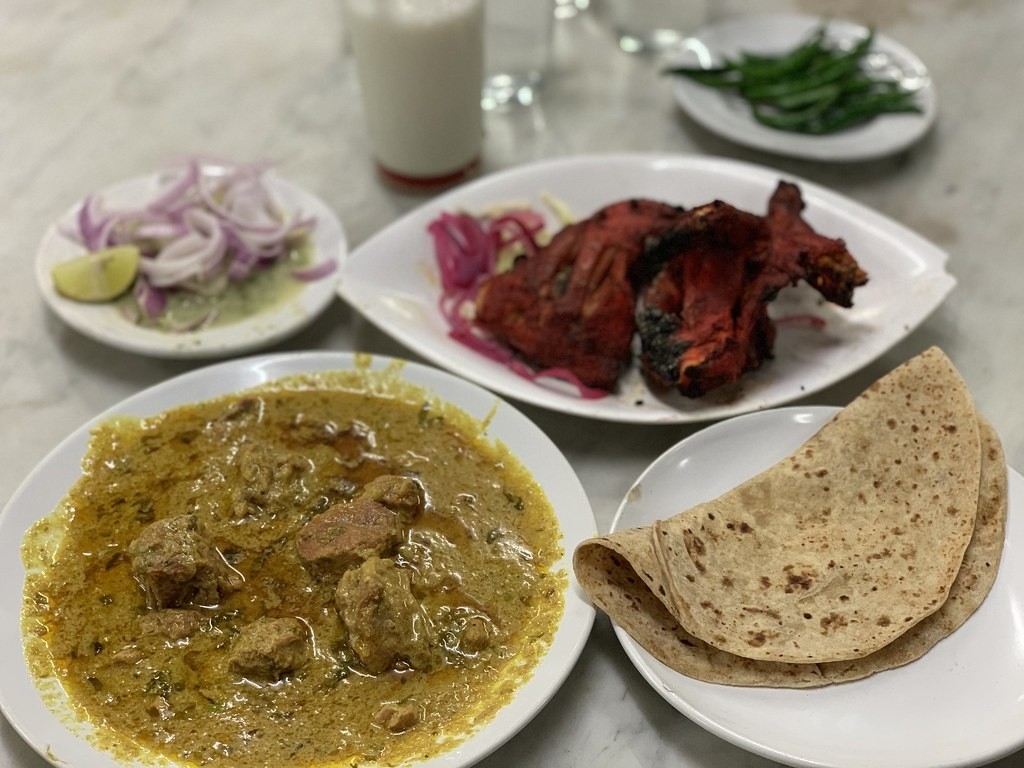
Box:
[337,2,705,197]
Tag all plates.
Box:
[330,150,957,426]
[33,167,343,356]
[604,404,1022,768]
[673,13,938,164]
[0,350,600,765]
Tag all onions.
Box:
[74,154,340,316]
